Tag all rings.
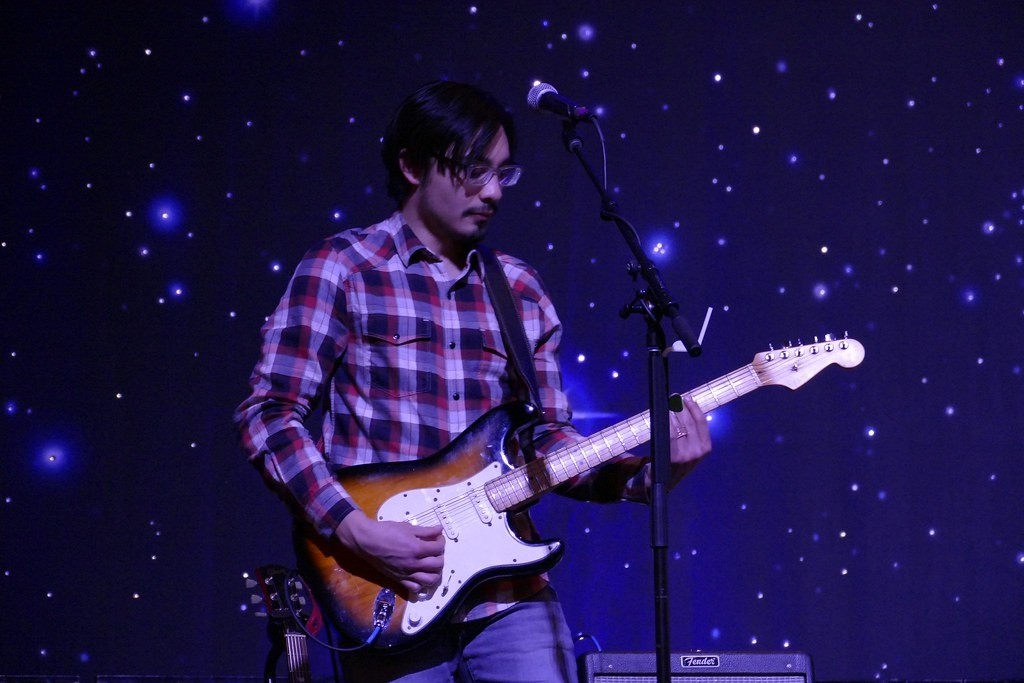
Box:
[676,432,688,439]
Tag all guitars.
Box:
[255,565,323,683]
[289,328,868,649]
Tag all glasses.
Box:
[431,153,529,186]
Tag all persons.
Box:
[232,75,712,683]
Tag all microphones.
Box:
[527,83,600,124]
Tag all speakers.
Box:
[576,651,809,683]
